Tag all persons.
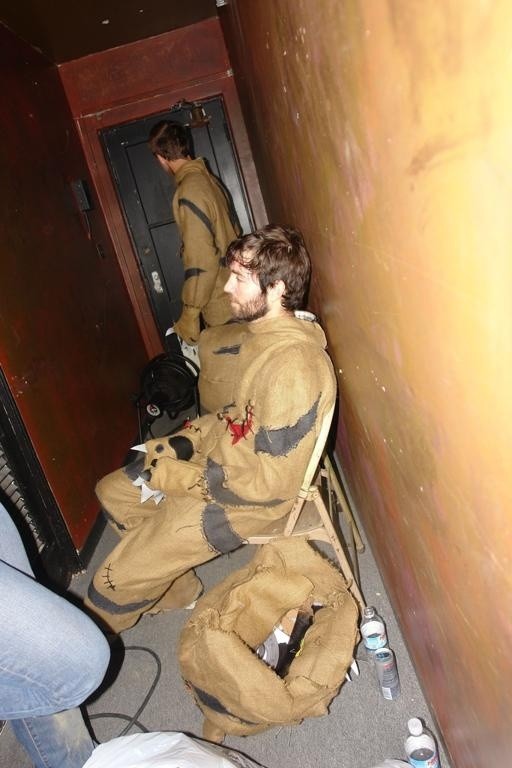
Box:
[77,222,338,640]
[0,501,114,766]
[147,121,242,379]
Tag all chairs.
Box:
[241,401,369,619]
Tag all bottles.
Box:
[359,606,389,670]
[404,717,439,767]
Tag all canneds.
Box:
[373,648,401,699]
